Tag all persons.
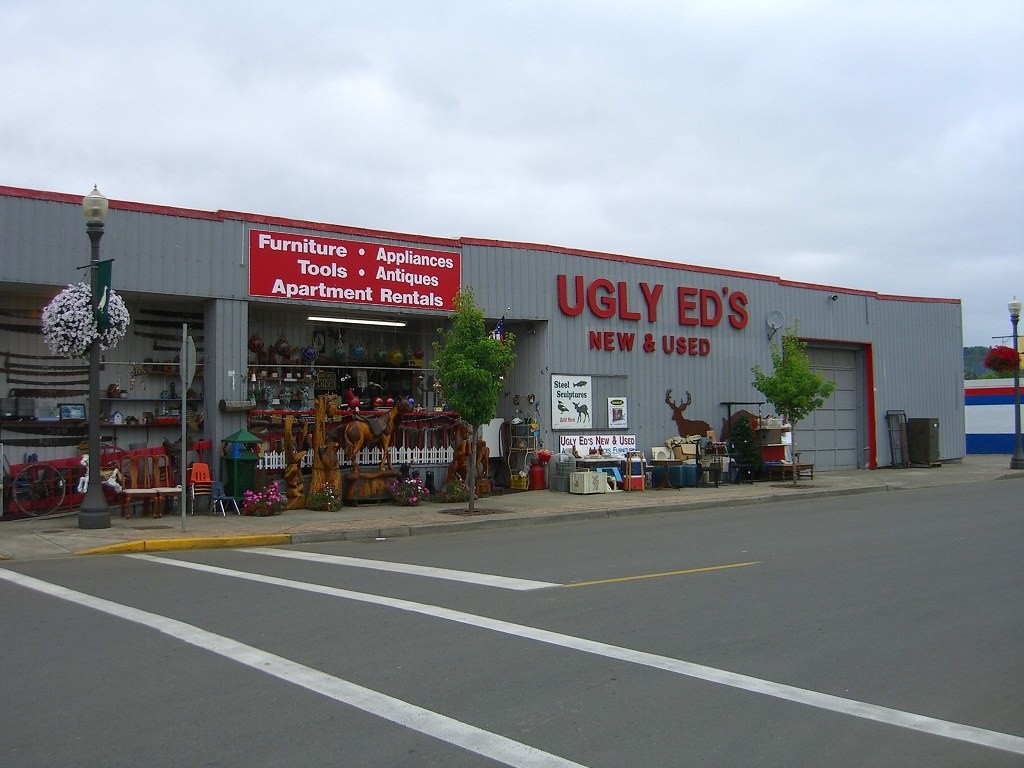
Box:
[267,385,277,409]
[283,387,291,409]
[299,387,310,408]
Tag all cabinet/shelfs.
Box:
[507,418,541,492]
[101,396,203,426]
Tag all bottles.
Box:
[589,446,603,455]
[572,446,576,455]
[706,443,725,454]
[757,414,788,426]
[627,452,644,459]
[783,430,792,444]
[562,448,565,453]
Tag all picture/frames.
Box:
[58,402,86,422]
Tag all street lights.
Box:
[1007,296,1024,469]
[78,183,112,530]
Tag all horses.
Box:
[343,395,414,473]
[78,454,126,493]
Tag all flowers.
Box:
[242,481,287,516]
[435,474,469,496]
[41,282,130,358]
[307,481,343,512]
[387,477,429,506]
[982,345,1020,371]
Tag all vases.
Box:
[320,503,328,511]
[998,360,1009,369]
[447,494,460,502]
[267,507,275,516]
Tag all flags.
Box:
[488,316,509,383]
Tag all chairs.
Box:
[121,453,241,517]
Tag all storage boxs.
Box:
[652,465,699,487]
[570,471,607,494]
[620,475,645,491]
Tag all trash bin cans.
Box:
[908,417,942,468]
[219,427,263,502]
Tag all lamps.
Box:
[306,311,408,327]
[825,294,838,303]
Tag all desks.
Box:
[626,451,645,493]
[769,464,815,482]
